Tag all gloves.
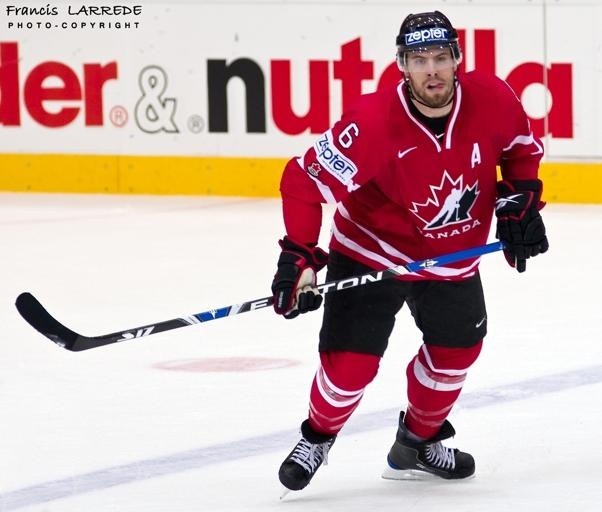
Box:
[270,234,329,320]
[495,177,548,274]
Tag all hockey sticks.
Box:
[15,242,506,351]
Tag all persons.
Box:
[271,9,550,490]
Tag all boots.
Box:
[388,412,475,479]
[280,421,336,489]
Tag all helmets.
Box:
[395,10,460,70]
